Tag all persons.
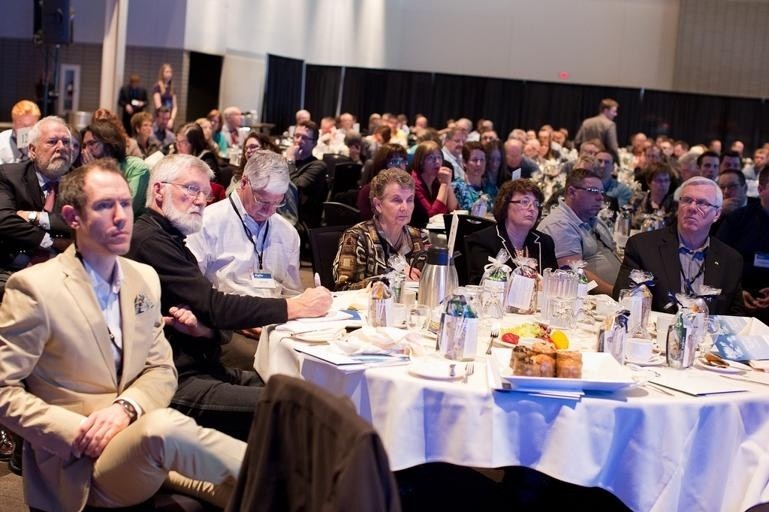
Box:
[574,99,622,172]
[207,109,228,153]
[66,123,80,163]
[711,160,769,326]
[561,139,601,178]
[92,108,144,160]
[152,63,178,132]
[657,132,769,180]
[463,177,559,286]
[503,139,540,181]
[0,115,76,301]
[332,168,432,292]
[524,138,540,160]
[224,131,282,199]
[451,140,499,213]
[312,116,336,160]
[536,125,573,164]
[184,150,302,371]
[281,120,328,228]
[484,140,504,197]
[612,176,746,316]
[222,106,245,150]
[0,157,248,512]
[634,145,679,195]
[124,153,335,442]
[630,162,679,230]
[536,168,623,297]
[411,141,460,227]
[445,117,495,131]
[627,132,647,155]
[356,143,409,220]
[195,117,219,160]
[80,119,151,217]
[336,109,443,165]
[152,106,177,148]
[710,169,761,237]
[160,122,221,183]
[594,150,634,207]
[275,109,311,149]
[0,100,41,165]
[509,128,528,155]
[441,128,467,184]
[130,112,160,160]
[117,73,150,138]
[478,128,498,146]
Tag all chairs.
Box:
[322,150,353,165]
[446,213,496,237]
[326,189,358,223]
[309,225,361,291]
[246,375,406,512]
[449,248,476,286]
[328,161,363,190]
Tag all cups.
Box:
[466,284,483,317]
[626,339,662,362]
[483,286,505,319]
[454,287,476,319]
[656,316,673,354]
[407,304,432,358]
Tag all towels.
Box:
[339,325,419,358]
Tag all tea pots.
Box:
[409,224,462,308]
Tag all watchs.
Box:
[27,211,39,224]
[114,398,138,425]
[287,159,295,165]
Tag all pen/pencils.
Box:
[314,273,320,288]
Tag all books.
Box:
[648,374,750,397]
[293,343,412,366]
[706,314,769,361]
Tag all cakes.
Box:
[529,354,555,378]
[513,357,541,377]
[529,341,557,360]
[510,346,536,369]
[556,358,581,378]
[556,349,583,365]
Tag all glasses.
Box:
[679,197,719,209]
[575,186,606,195]
[720,183,741,190]
[508,198,539,208]
[248,179,286,207]
[389,159,409,167]
[161,181,215,202]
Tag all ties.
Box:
[27,185,56,268]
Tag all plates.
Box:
[632,357,666,367]
[411,361,465,380]
[492,347,634,391]
[698,358,752,373]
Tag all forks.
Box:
[486,330,499,354]
[464,362,475,383]
